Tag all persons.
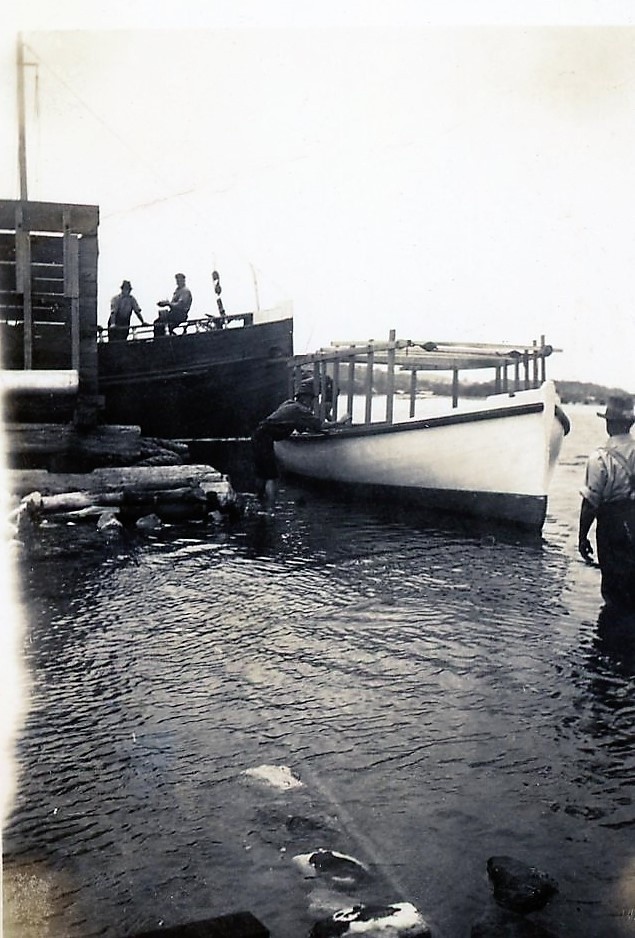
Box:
[579,395,635,641]
[154,273,192,337]
[107,280,148,341]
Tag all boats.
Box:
[97,271,298,436]
[270,330,570,534]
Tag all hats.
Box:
[121,281,131,290]
[293,386,315,399]
[596,393,635,422]
